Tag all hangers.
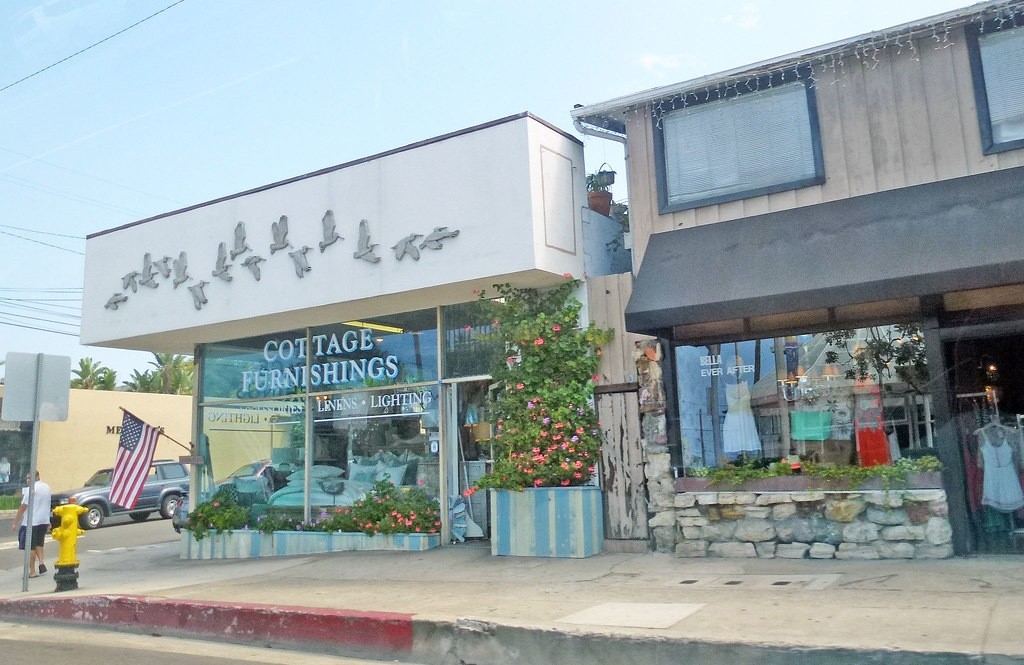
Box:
[972,413,1020,436]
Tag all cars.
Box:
[170,460,288,529]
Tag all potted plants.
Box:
[180,473,442,561]
[598,162,618,187]
[586,173,612,217]
[609,200,628,217]
[463,272,603,559]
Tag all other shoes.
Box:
[38,564,47,574]
[28,573,39,578]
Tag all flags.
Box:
[109,411,160,510]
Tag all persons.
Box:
[10,470,52,578]
[852,370,891,467]
[722,381,762,452]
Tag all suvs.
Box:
[49,455,193,531]
